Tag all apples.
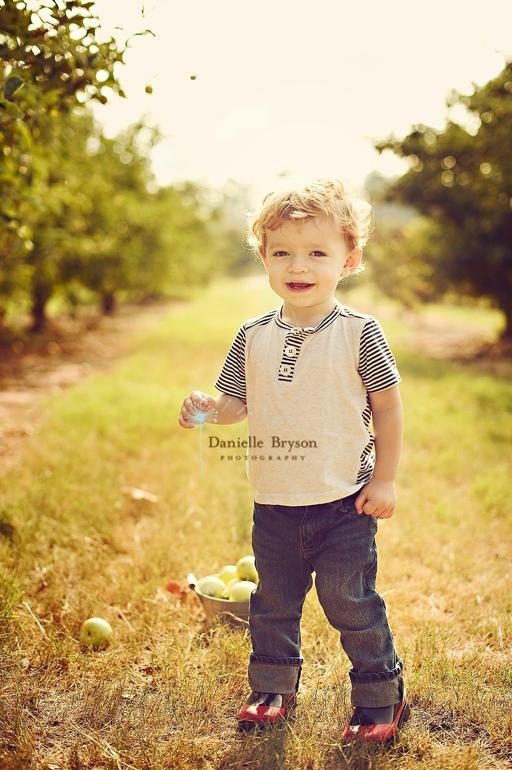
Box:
[79,617,113,648]
[197,555,259,602]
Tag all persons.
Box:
[176,176,411,747]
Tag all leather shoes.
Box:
[238,693,300,727]
[341,701,411,746]
